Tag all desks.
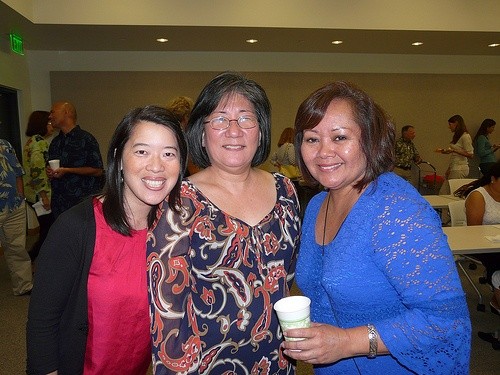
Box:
[422,195,466,208]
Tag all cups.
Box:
[274,295,312,353]
[49,160,60,174]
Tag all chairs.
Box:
[448,200,483,266]
[416,161,445,195]
[446,179,479,227]
[442,225,500,299]
